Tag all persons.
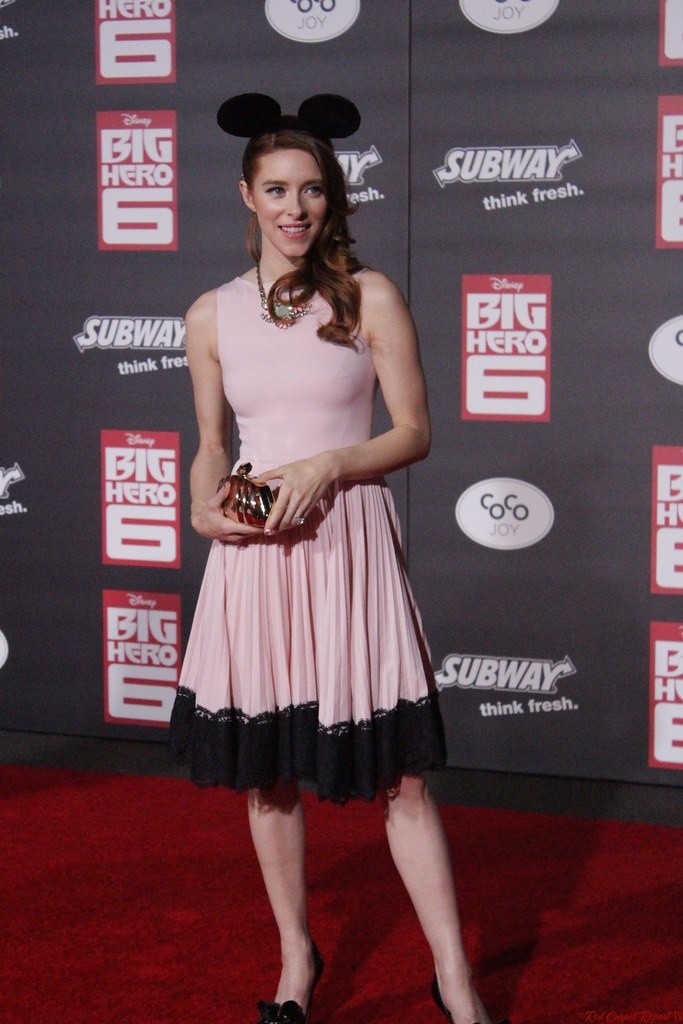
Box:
[170,120,492,1023]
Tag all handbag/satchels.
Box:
[216,462,275,528]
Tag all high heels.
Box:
[431,972,510,1024]
[256,941,324,1024]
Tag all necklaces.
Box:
[256,254,312,329]
